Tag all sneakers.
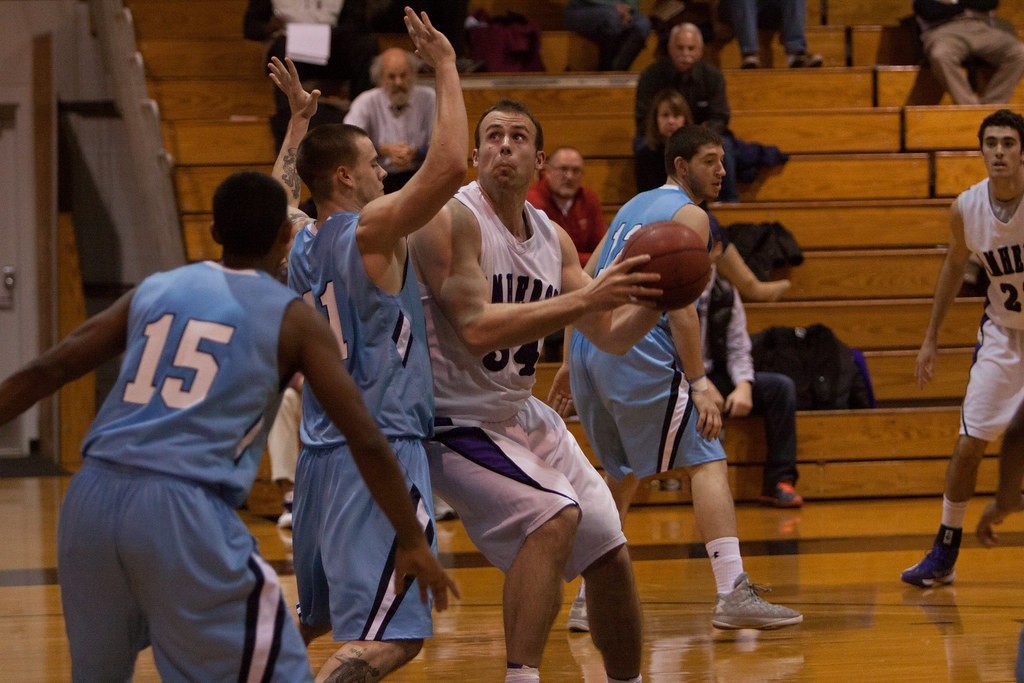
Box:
[711,572,803,629]
[902,547,956,586]
[714,648,805,683]
[567,632,603,666]
[759,475,803,506]
[568,595,591,631]
[922,588,964,638]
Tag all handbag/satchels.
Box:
[754,324,875,406]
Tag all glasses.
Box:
[547,164,583,177]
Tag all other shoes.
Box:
[434,492,454,520]
[277,499,292,526]
[741,53,760,67]
[789,52,823,68]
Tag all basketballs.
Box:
[621,221,712,309]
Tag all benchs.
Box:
[126,0,1023,518]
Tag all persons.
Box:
[635,88,792,303]
[341,46,436,191]
[526,145,608,249]
[407,99,670,683]
[902,108,1024,681]
[696,226,802,508]
[240,0,824,112]
[911,0,1024,105]
[268,6,468,683]
[546,123,803,632]
[0,169,461,683]
[636,22,743,202]
